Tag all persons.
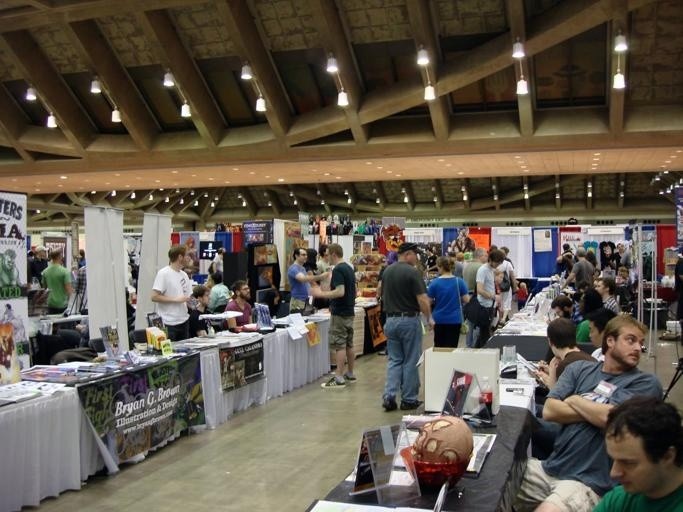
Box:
[376,243,528,412]
[303,248,322,311]
[236,367,247,386]
[308,243,358,390]
[588,394,683,511]
[511,313,664,512]
[287,248,329,314]
[250,288,280,324]
[673,250,682,321]
[26,245,254,341]
[532,237,653,461]
[448,226,475,252]
[314,243,334,286]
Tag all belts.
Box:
[384,311,421,318]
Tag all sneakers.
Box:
[498,320,505,328]
[343,374,358,383]
[319,376,346,389]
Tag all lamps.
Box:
[240,60,267,112]
[163,67,193,118]
[511,36,529,96]
[90,75,122,123]
[611,28,629,89]
[416,43,436,102]
[25,87,58,130]
[325,49,349,107]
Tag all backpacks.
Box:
[495,262,511,294]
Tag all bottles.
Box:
[479,375,494,423]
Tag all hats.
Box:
[398,242,425,255]
[35,245,50,253]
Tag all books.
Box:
[0,336,231,406]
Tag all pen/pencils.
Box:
[539,360,549,371]
[547,312,550,320]
[115,359,128,363]
[459,486,465,501]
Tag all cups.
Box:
[502,346,517,364]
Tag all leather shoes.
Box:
[381,398,398,412]
[399,398,424,411]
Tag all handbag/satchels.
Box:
[460,317,470,336]
[462,292,497,333]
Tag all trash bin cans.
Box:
[643,302,667,329]
